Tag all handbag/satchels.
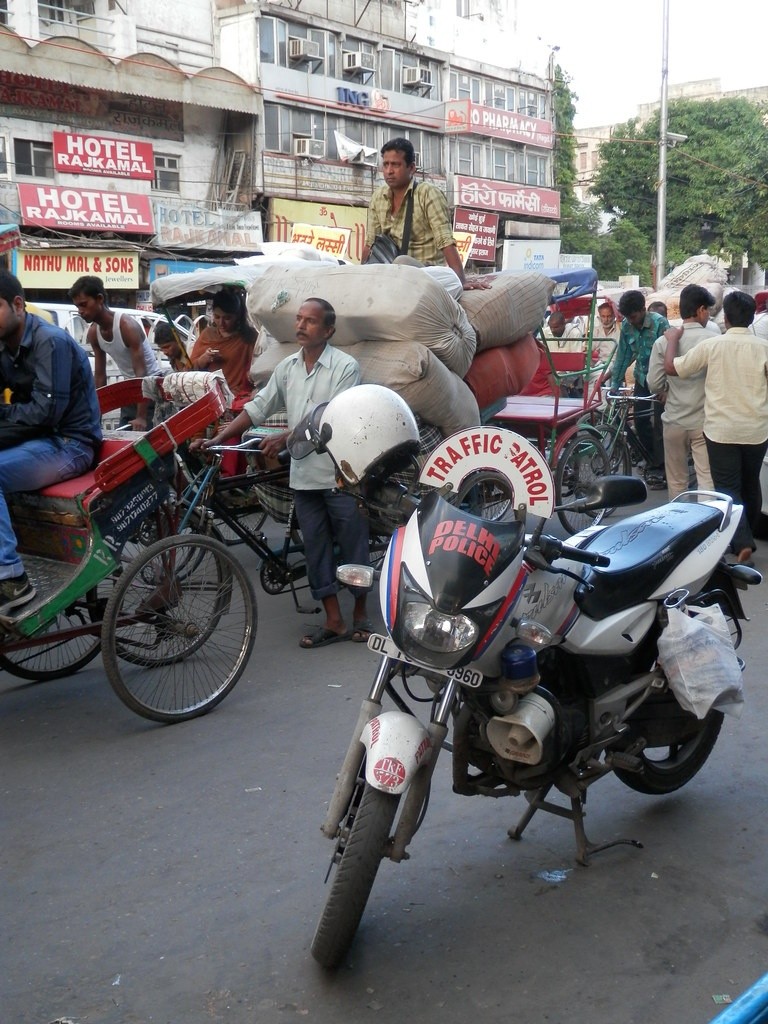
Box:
[363,233,401,265]
[658,599,744,721]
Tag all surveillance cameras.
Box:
[667,132,688,142]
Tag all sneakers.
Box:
[0,574,36,611]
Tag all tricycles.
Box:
[487,269,616,491]
[0,369,234,680]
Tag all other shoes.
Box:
[645,474,668,491]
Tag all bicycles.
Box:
[101,438,424,724]
[555,390,664,535]
[595,387,644,466]
[104,424,271,546]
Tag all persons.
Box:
[189,299,373,647]
[0,268,103,608]
[664,291,768,563]
[362,137,492,290]
[67,275,162,431]
[542,313,583,397]
[185,288,283,478]
[646,284,720,502]
[610,291,670,483]
[651,301,667,319]
[591,301,622,387]
[748,292,768,340]
[155,322,193,372]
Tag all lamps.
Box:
[463,13,484,21]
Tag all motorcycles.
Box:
[274,383,761,967]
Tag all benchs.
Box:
[37,467,104,526]
[493,353,597,424]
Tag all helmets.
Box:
[319,384,421,485]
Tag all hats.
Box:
[155,323,178,342]
[754,292,768,312]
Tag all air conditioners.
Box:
[403,67,433,86]
[342,52,375,72]
[294,139,325,158]
[289,40,319,57]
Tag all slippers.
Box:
[724,544,755,568]
[300,627,350,647]
[353,619,374,641]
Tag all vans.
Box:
[24,302,170,387]
